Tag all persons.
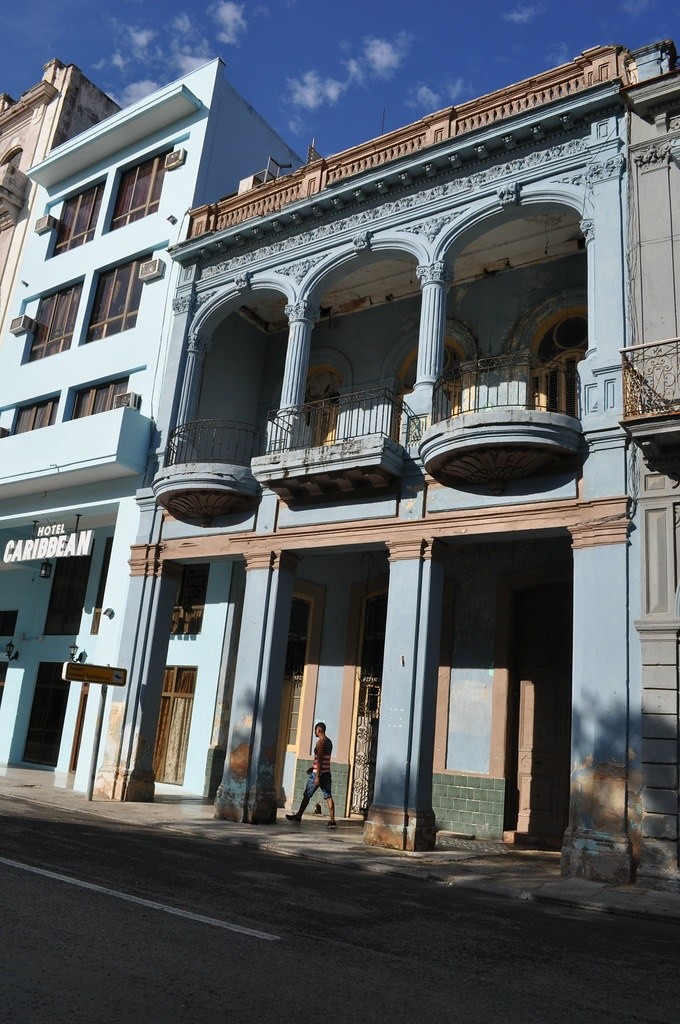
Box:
[285,723,337,827]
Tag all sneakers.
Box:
[285,814,302,825]
[325,821,337,828]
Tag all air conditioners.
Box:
[112,392,143,414]
[163,147,188,173]
[8,313,38,339]
[34,214,59,237]
[138,257,167,286]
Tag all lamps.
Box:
[5,640,18,662]
[40,561,53,579]
[103,608,115,619]
[68,641,79,662]
